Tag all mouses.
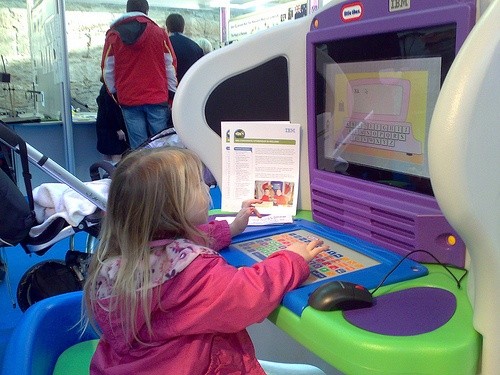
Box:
[308,281,374,312]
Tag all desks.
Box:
[174,0,499,374]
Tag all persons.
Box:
[95,77,130,162]
[166,13,205,134]
[98,0,179,149]
[65,144,330,375]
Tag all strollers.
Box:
[0,119,217,312]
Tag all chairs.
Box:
[2,291,104,375]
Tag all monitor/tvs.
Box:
[307,0,477,267]
